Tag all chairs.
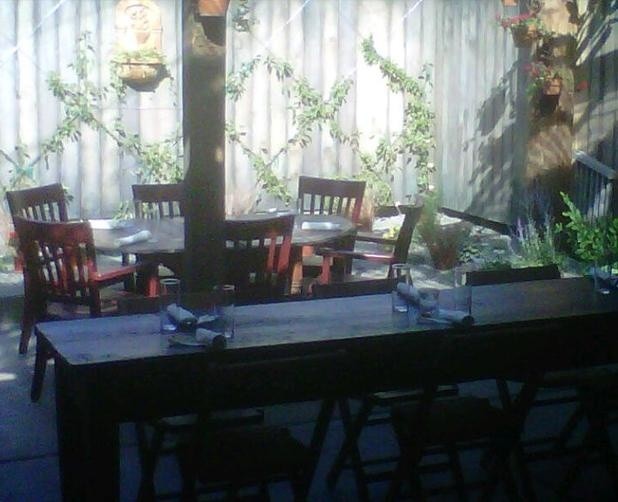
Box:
[7,180,70,234]
[205,212,297,298]
[294,175,368,278]
[10,214,144,356]
[112,263,618,502]
[129,181,193,219]
[311,203,422,299]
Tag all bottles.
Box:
[417,288,439,319]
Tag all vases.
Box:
[510,26,535,48]
[543,77,562,98]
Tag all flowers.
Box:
[523,59,565,106]
[495,11,546,35]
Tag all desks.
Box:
[53,209,361,295]
[32,274,617,502]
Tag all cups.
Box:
[594,258,611,295]
[453,267,470,286]
[391,264,410,312]
[160,278,181,331]
[212,283,236,338]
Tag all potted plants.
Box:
[111,47,168,87]
